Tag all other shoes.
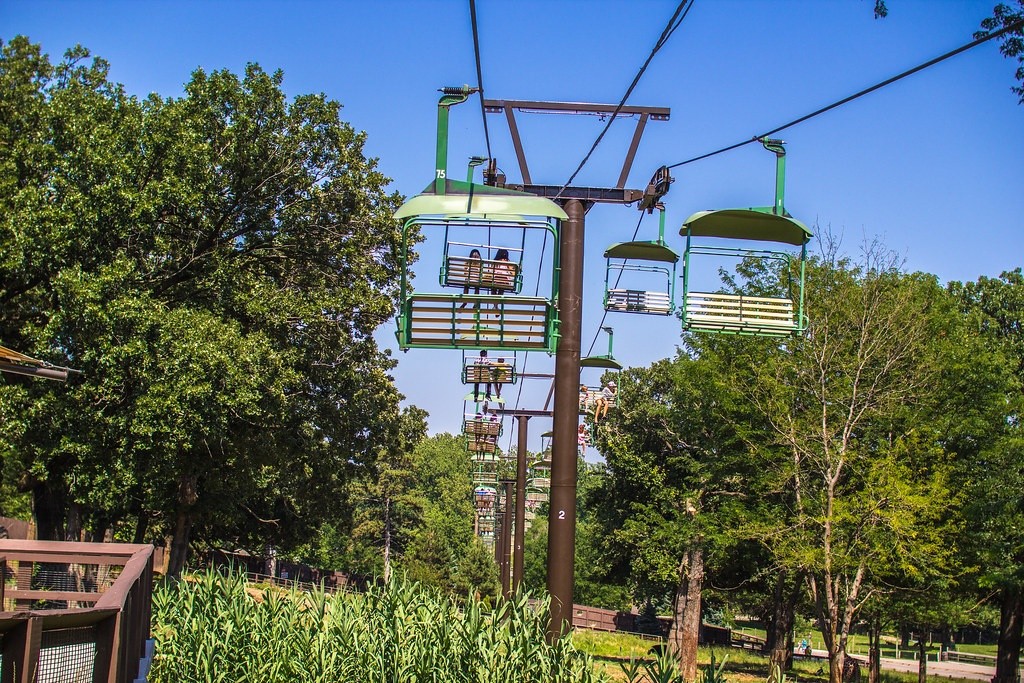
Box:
[474,395,493,403]
[592,416,607,426]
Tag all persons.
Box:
[484,414,497,444]
[490,249,510,318]
[476,434,496,461]
[492,358,505,399]
[578,424,591,456]
[460,249,481,309]
[593,381,617,425]
[474,413,483,442]
[473,350,510,402]
[480,488,490,512]
[579,386,589,412]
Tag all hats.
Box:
[607,381,617,386]
